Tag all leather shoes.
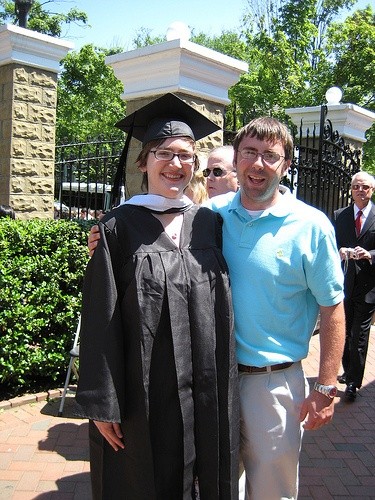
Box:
[345,385,357,399]
[339,372,347,383]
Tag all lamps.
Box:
[325,87,343,105]
[165,21,193,41]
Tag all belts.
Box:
[239,362,293,373]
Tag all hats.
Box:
[109,92,221,208]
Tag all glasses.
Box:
[149,150,198,162]
[352,185,373,191]
[202,167,235,177]
[238,149,286,163]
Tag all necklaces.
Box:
[159,213,183,240]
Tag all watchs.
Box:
[313,382,338,399]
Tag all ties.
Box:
[355,211,361,237]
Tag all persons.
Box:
[87,118,347,500]
[202,145,240,198]
[77,113,241,500]
[332,172,375,399]
